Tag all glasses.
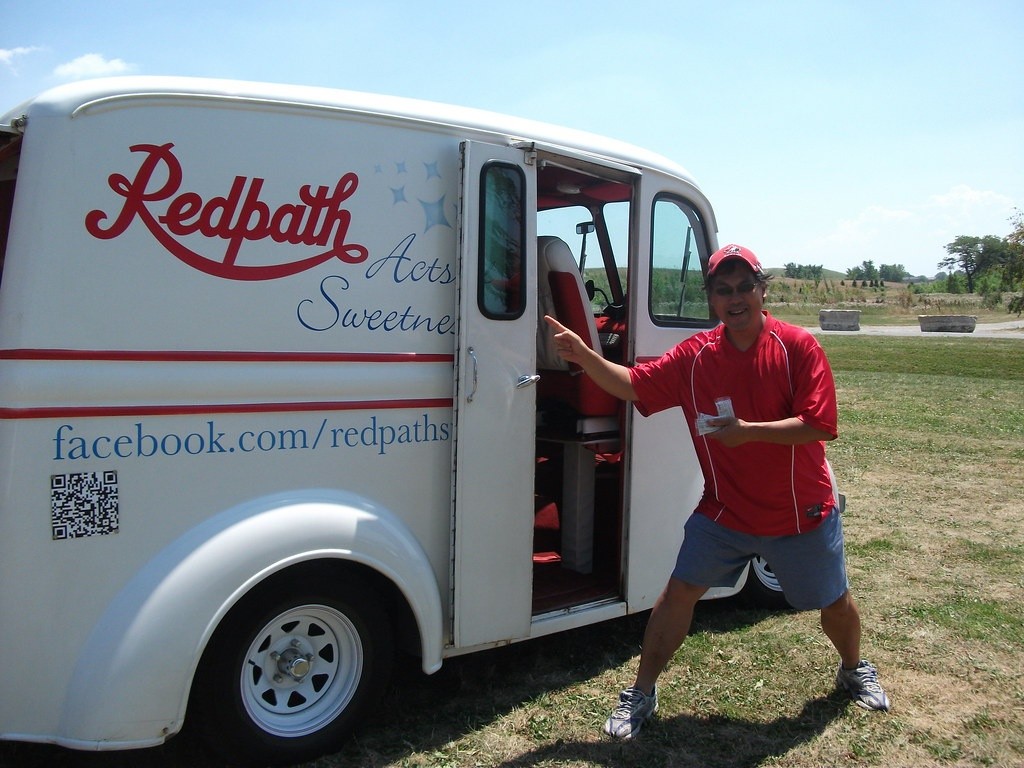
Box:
[710,281,760,296]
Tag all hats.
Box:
[705,243,764,278]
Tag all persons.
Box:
[544,248,893,740]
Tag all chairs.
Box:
[538,236,622,575]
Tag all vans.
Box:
[0,80,848,768]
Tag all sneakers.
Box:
[835,659,889,711]
[605,681,659,739]
